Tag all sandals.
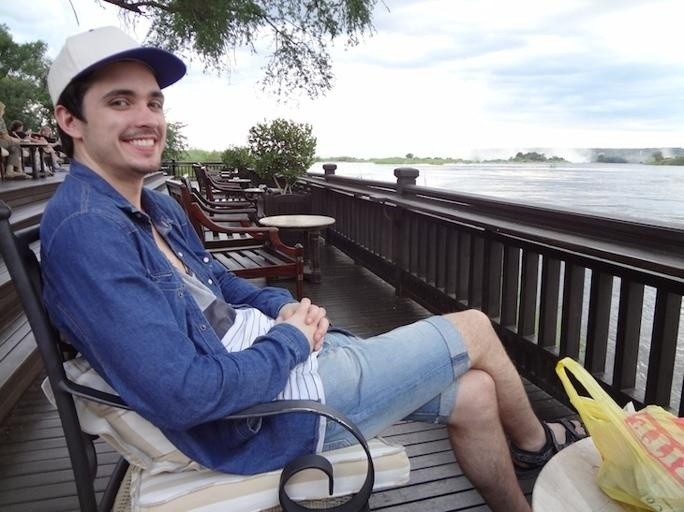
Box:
[509,414,590,480]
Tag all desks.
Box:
[531,434,630,512]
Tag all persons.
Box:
[0,102,70,181]
[37,27,590,512]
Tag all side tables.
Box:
[244,186,283,213]
[258,214,336,281]
[228,177,252,186]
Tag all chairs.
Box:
[1,196,410,512]
[164,162,305,303]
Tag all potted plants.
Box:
[246,117,318,246]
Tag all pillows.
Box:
[39,356,207,473]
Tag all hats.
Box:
[46,24,188,111]
